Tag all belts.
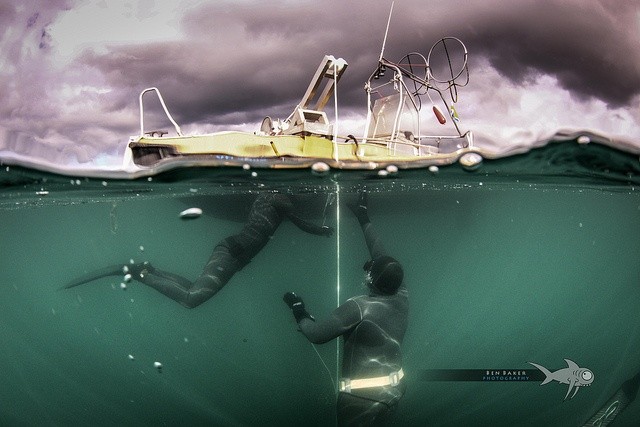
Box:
[338,368,404,391]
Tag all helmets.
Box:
[364,254,404,295]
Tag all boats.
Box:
[128,36,484,223]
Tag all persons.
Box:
[58,179,335,308]
[284,191,412,426]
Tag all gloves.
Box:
[346,191,371,227]
[321,225,336,238]
[282,290,306,319]
